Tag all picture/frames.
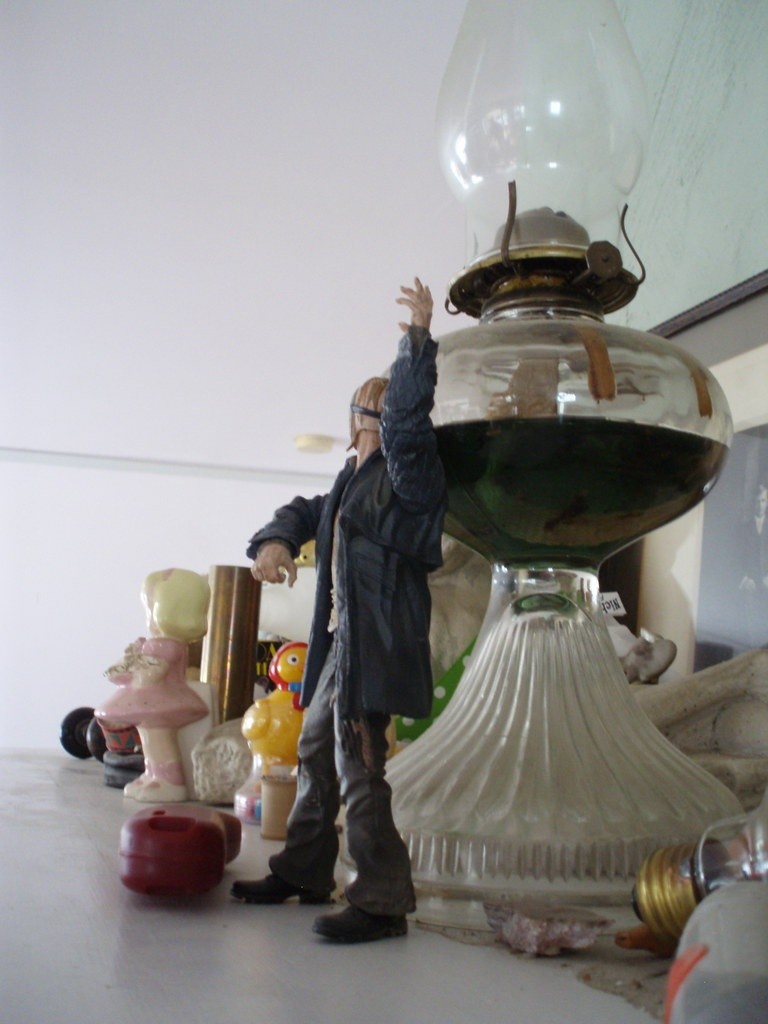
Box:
[613,267,768,686]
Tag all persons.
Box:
[230,277,444,942]
[94,567,211,803]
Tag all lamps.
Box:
[335,0,747,907]
